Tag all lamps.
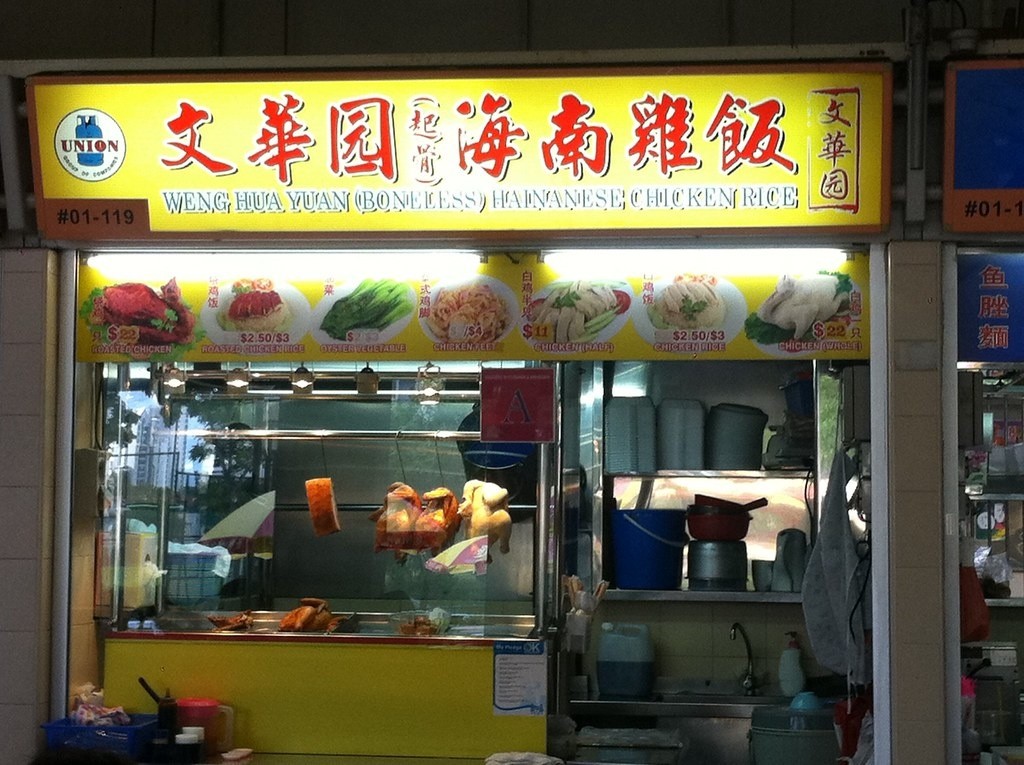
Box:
[415,363,443,403]
[291,362,313,393]
[164,371,187,397]
[227,368,250,393]
[353,362,379,394]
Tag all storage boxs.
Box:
[40,713,158,761]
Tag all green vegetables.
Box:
[320,277,414,342]
[743,270,853,345]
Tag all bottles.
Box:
[158,688,178,730]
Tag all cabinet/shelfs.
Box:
[559,357,854,718]
[950,364,1024,606]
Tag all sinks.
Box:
[658,693,778,717]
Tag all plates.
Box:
[418,274,518,347]
[633,277,746,354]
[603,396,769,470]
[309,281,417,347]
[751,277,860,356]
[518,279,634,353]
[200,282,311,356]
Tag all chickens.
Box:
[369,479,513,570]
[205,608,256,636]
[757,273,851,341]
[96,274,196,349]
[277,596,362,634]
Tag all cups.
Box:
[176,726,206,765]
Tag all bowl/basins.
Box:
[685,540,749,582]
[790,691,822,710]
[752,559,774,591]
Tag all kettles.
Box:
[771,528,809,592]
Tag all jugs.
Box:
[176,698,233,765]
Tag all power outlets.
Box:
[980,646,1017,667]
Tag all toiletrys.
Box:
[778,631,804,697]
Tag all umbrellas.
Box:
[196,491,275,561]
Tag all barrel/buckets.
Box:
[597,622,654,698]
[611,508,687,590]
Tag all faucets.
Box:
[730,622,755,696]
